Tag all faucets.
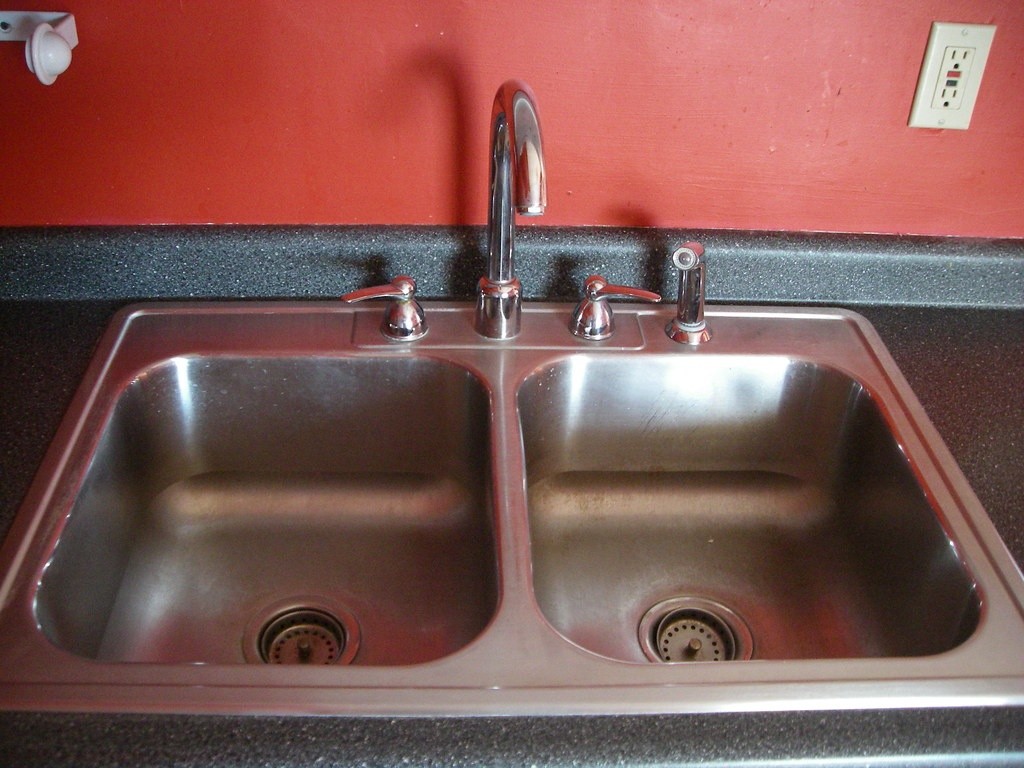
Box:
[471,79,550,339]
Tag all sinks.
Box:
[504,296,1024,721]
[0,298,504,720]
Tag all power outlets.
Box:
[907,22,997,131]
